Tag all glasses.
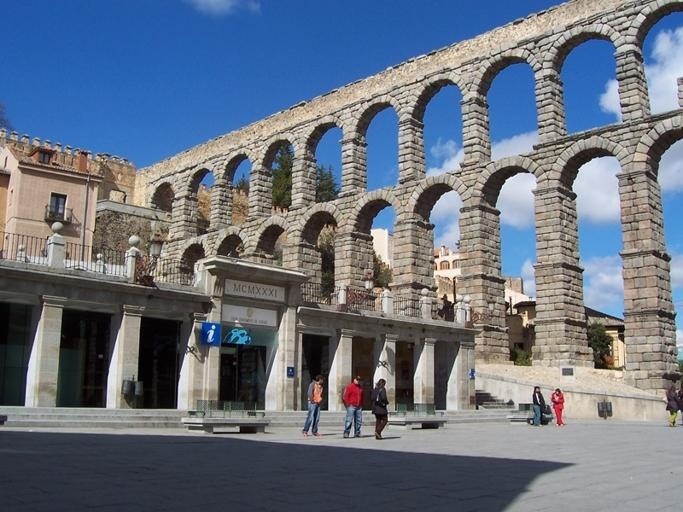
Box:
[355,378,361,380]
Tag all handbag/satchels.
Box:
[376,388,385,406]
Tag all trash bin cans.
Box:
[598,399,612,420]
[121,375,143,408]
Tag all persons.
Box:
[551,388,565,426]
[664,383,681,427]
[678,381,683,426]
[301,374,324,439]
[371,378,389,440]
[532,386,545,426]
[340,373,365,439]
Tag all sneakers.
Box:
[535,425,543,427]
[556,424,563,426]
[355,435,360,438]
[344,434,349,438]
[313,432,320,437]
[303,432,308,437]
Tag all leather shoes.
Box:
[375,432,383,439]
